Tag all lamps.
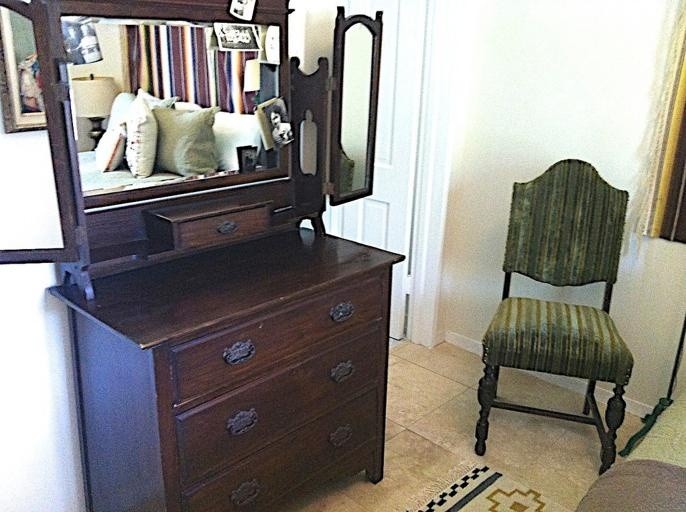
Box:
[72,72,121,150]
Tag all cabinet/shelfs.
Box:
[48,199,408,512]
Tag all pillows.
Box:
[94,91,262,177]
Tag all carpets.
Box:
[413,459,576,512]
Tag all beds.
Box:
[76,151,184,193]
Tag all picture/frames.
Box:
[0,5,49,133]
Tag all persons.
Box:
[65,25,101,64]
[266,105,293,146]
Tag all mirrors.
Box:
[334,21,372,200]
[0,10,67,257]
[58,14,291,197]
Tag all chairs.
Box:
[473,160,637,480]
